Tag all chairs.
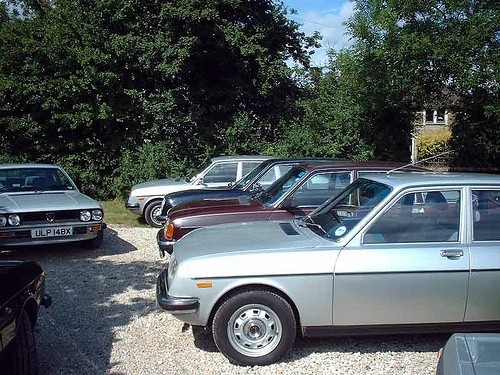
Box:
[410,192,453,240]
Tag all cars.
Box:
[157,160,500,270]
[124,155,341,228]
[157,158,350,228]
[0,255,53,375]
[155,149,500,368]
[0,164,107,261]
